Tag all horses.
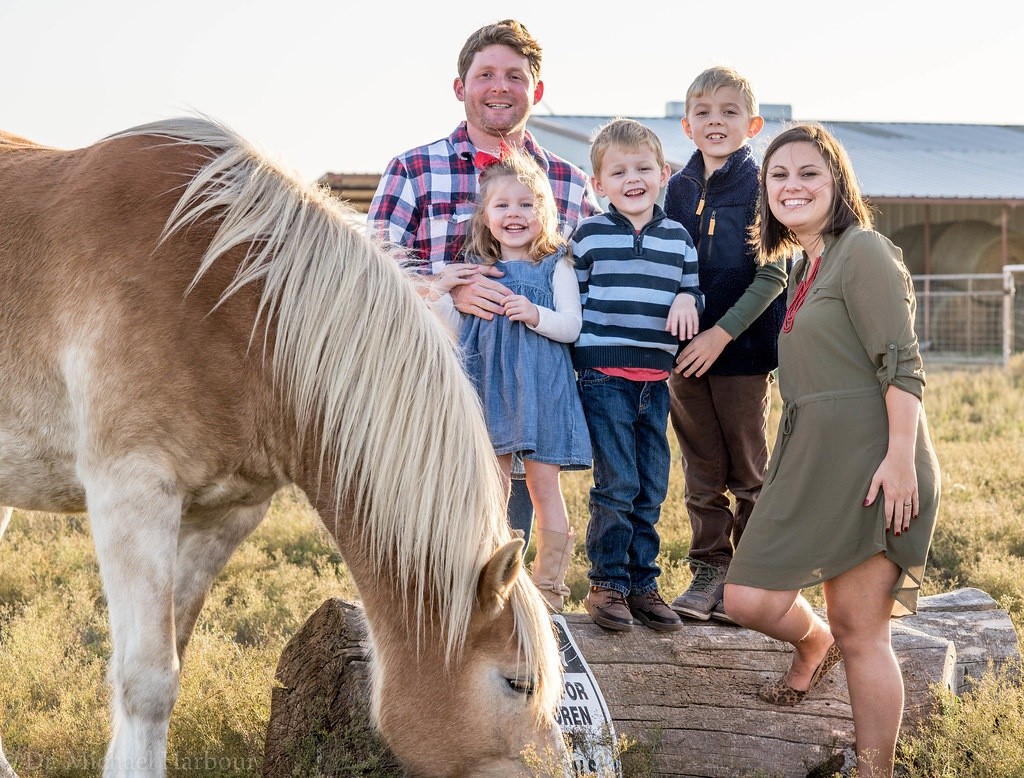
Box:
[0,115,574,777]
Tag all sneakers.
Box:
[584,586,633,632]
[628,589,683,632]
[712,599,745,628]
[671,557,729,620]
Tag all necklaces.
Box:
[782,246,824,333]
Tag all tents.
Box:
[522,108,1024,342]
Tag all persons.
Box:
[722,121,945,778]
[364,19,606,564]
[564,117,707,634]
[664,65,792,619]
[423,142,582,619]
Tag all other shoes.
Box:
[759,643,844,705]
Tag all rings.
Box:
[904,504,913,506]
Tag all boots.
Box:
[531,528,576,614]
